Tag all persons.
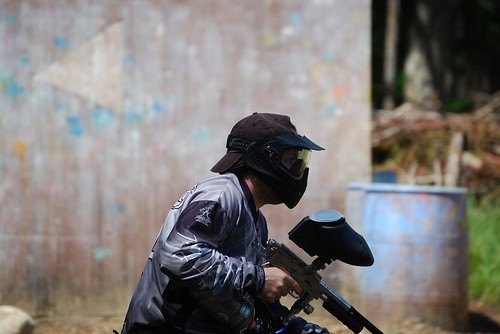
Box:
[121,113,334,334]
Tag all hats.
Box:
[210,111,301,172]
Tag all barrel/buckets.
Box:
[339,182,369,313]
[358,184,470,334]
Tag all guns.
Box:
[250,209,385,334]
